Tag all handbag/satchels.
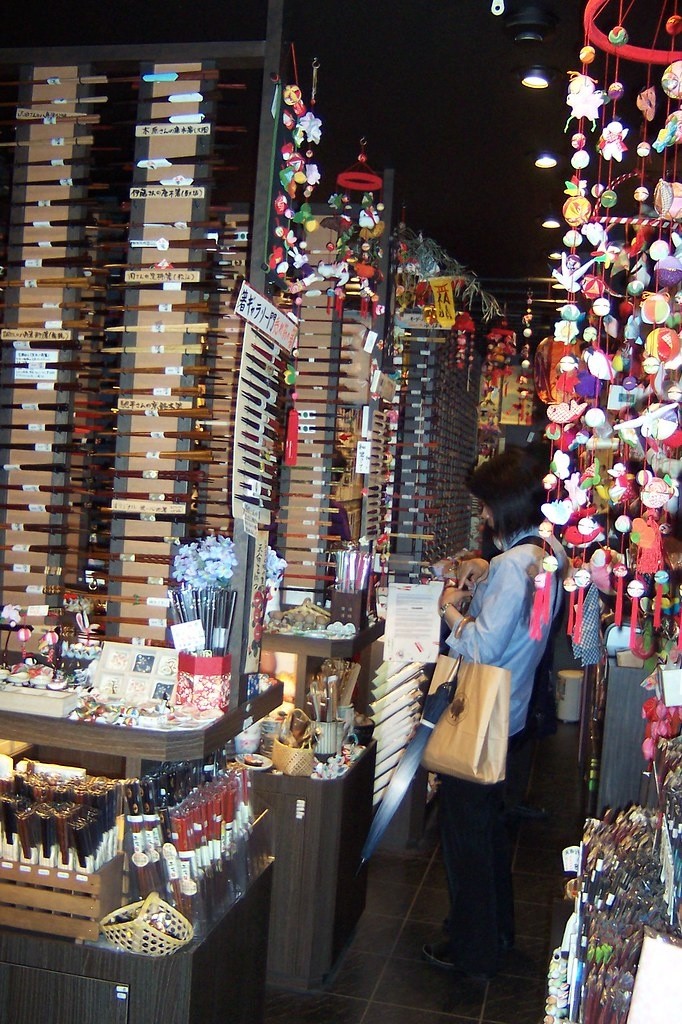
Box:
[418,628,510,784]
[526,639,558,736]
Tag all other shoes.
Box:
[422,941,503,972]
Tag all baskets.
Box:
[101,891,194,958]
[272,709,314,777]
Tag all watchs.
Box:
[438,603,452,618]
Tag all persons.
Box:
[423,444,569,971]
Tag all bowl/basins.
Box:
[350,719,375,746]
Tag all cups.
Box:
[337,721,345,754]
[316,722,337,761]
[337,708,354,737]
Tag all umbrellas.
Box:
[355,616,476,875]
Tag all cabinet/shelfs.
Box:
[0,662,284,1024]
